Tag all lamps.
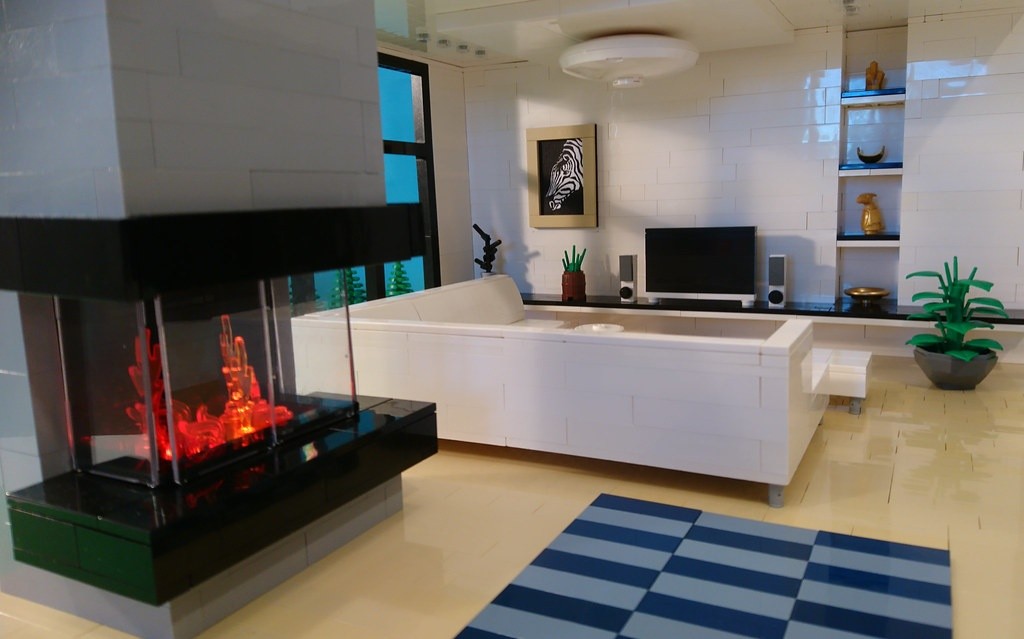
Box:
[560,34,699,89]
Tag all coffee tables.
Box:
[832,351,873,414]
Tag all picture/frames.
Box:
[526,123,598,228]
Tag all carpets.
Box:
[454,492,953,639]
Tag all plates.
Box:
[575,323,626,336]
[844,287,891,304]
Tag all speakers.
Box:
[620,255,637,304]
[768,255,787,309]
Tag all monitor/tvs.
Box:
[644,226,758,308]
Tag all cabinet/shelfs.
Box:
[836,88,907,248]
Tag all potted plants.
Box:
[561,245,587,303]
[905,256,1008,392]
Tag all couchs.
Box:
[288,274,831,509]
